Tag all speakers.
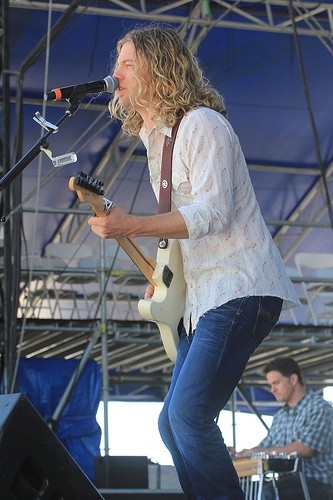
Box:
[0,393,104,500]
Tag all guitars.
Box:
[65,169,186,363]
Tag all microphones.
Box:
[44,76,118,101]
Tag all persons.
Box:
[88,26,303,500]
[235,356,333,500]
